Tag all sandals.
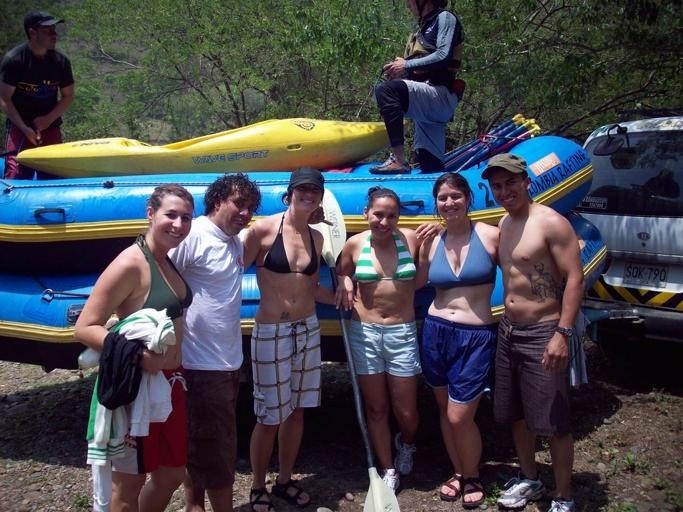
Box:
[272,478,310,507]
[462,478,485,507]
[440,474,462,500]
[250,488,273,512]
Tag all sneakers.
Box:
[394,432,416,475]
[382,468,400,494]
[370,153,410,173]
[548,496,574,512]
[497,471,545,509]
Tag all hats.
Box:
[482,154,527,179]
[24,12,64,27]
[290,167,323,188]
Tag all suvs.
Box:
[569,117,683,338]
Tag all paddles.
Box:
[307,186,405,512]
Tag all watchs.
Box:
[557,326,575,337]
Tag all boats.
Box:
[0,136,603,240]
[12,116,429,176]
[0,216,604,371]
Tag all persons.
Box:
[331,187,443,495]
[415,172,499,509]
[367,0,466,173]
[70,183,193,512]
[481,153,583,512]
[1,9,76,181]
[240,165,355,512]
[105,171,262,511]
